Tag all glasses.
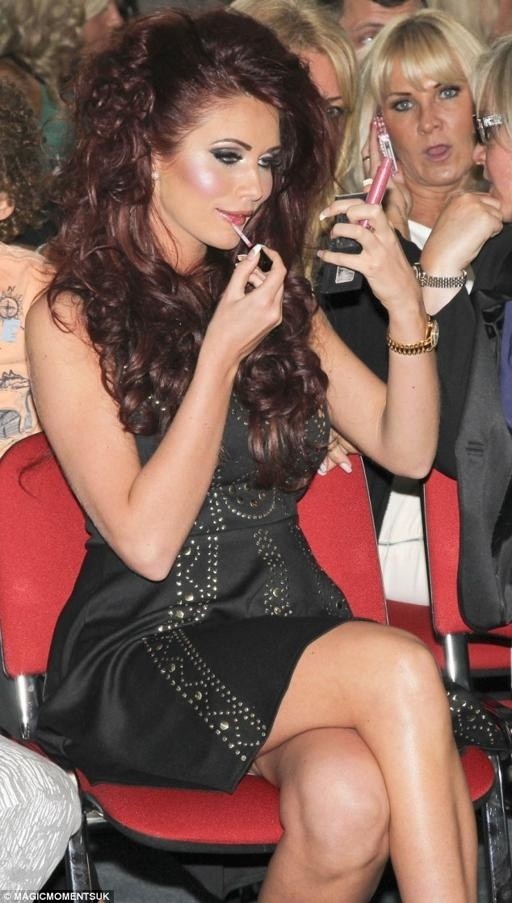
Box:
[472,112,510,144]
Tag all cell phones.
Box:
[379,133,398,176]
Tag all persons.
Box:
[228,0,362,278]
[0,0,89,130]
[412,45,512,635]
[20,0,511,903]
[2,62,90,447]
[82,0,124,62]
[325,2,444,63]
[317,9,496,663]
[423,0,511,44]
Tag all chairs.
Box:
[0,404,496,903]
[419,438,509,769]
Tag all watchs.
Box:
[385,315,440,359]
[413,260,467,290]
